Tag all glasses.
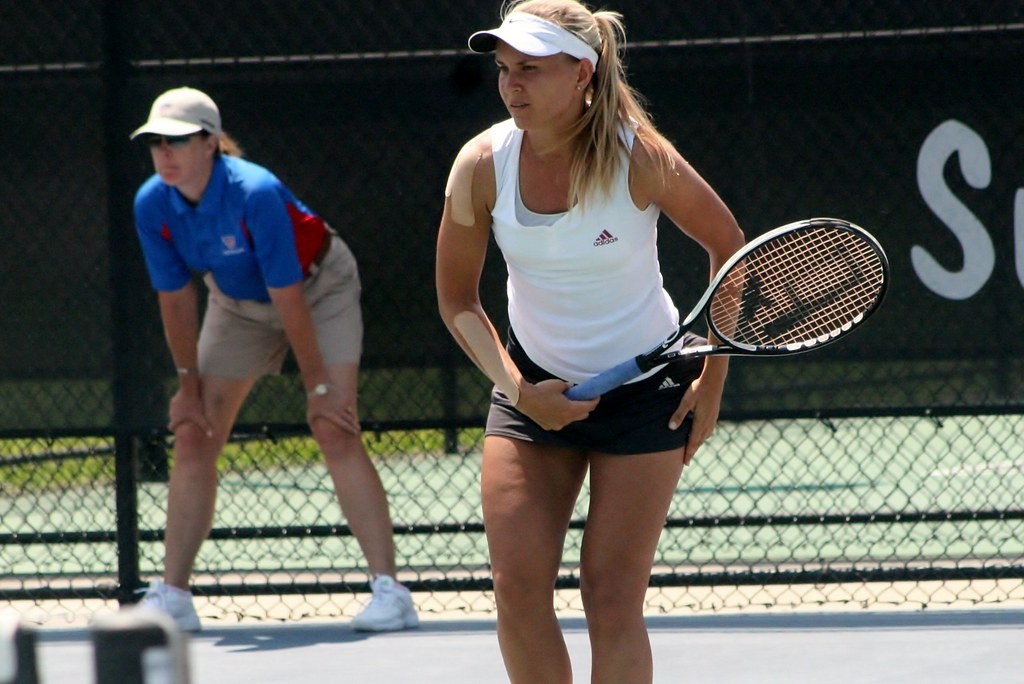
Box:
[144,133,205,148]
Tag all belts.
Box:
[303,229,335,282]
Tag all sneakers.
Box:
[134,580,201,631]
[349,574,419,630]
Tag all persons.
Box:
[130,87,419,634]
[434,0,747,684]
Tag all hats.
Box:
[468,11,598,72]
[129,86,221,140]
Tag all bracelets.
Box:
[176,367,198,376]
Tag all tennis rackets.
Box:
[561,216,891,401]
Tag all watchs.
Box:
[306,380,333,399]
